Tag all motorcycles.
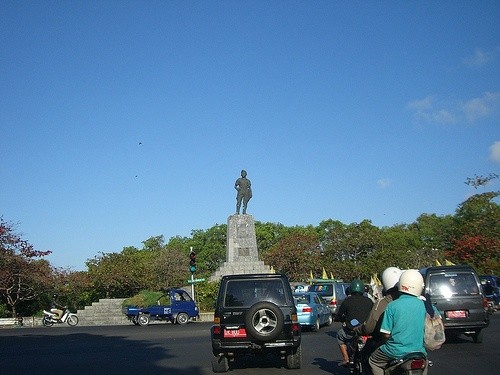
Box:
[331,313,373,375]
[350,320,434,375]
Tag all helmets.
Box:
[350,280,365,294]
[397,269,424,297]
[381,267,403,291]
[52,294,58,299]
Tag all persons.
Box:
[235,170,252,214]
[334,267,443,375]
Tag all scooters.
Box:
[42,306,79,327]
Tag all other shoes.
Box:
[338,360,349,366]
[58,319,62,323]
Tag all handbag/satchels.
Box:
[423,300,445,351]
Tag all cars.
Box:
[292,292,332,332]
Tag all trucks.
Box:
[121,288,201,327]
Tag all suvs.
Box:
[418,265,489,337]
[309,279,347,316]
[478,275,500,311]
[210,273,303,372]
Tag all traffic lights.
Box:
[190,252,197,273]
